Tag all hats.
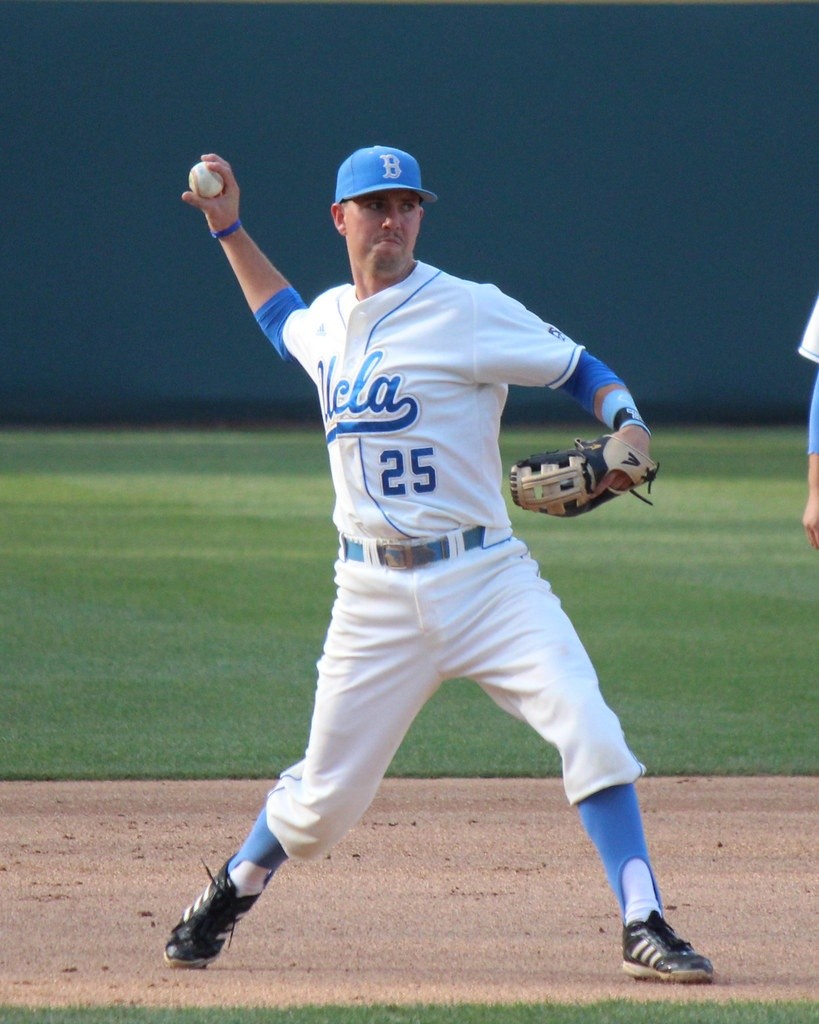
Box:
[333,145,438,205]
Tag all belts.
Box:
[343,527,486,572]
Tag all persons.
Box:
[165,142,714,982]
[796,272,819,550]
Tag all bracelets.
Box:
[205,219,246,239]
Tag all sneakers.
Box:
[620,909,714,982]
[162,853,262,971]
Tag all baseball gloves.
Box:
[510,432,658,520]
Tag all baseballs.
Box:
[189,162,224,198]
